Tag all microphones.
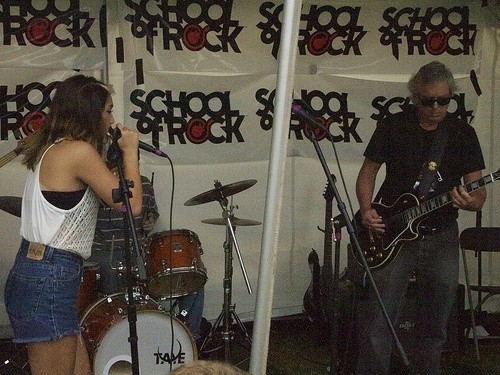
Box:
[292,104,328,132]
[112,127,169,157]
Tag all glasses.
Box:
[417,96,451,107]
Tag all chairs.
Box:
[460,227,500,362]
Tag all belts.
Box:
[421,223,445,235]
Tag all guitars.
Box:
[348,165,500,265]
[0,126,40,172]
[301,174,353,330]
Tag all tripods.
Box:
[197,197,251,366]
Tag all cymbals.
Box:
[184,178,259,205]
[201,218,262,227]
[0,194,25,220]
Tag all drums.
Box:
[72,293,197,375]
[138,228,205,297]
[74,262,99,321]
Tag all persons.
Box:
[356,62,486,375]
[168,361,248,375]
[94,143,204,341]
[4,75,143,375]
